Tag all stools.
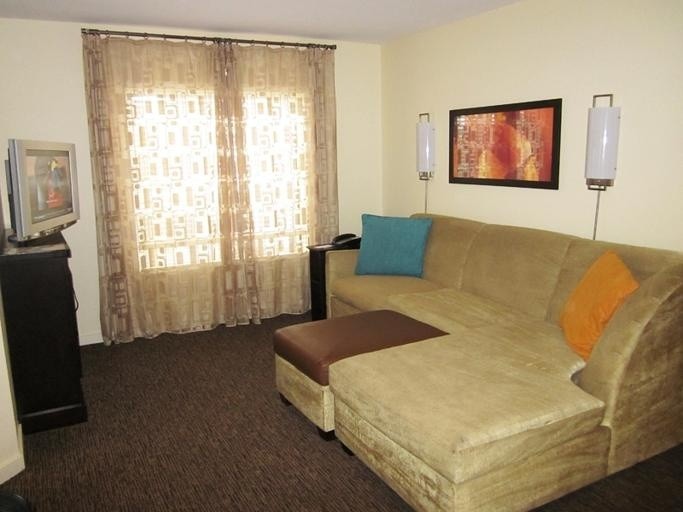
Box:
[272,308,450,441]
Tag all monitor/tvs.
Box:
[5,137,80,245]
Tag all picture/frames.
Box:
[447,97,562,191]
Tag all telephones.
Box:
[332,233,361,247]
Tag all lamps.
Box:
[415,113,436,214]
[583,93,621,240]
